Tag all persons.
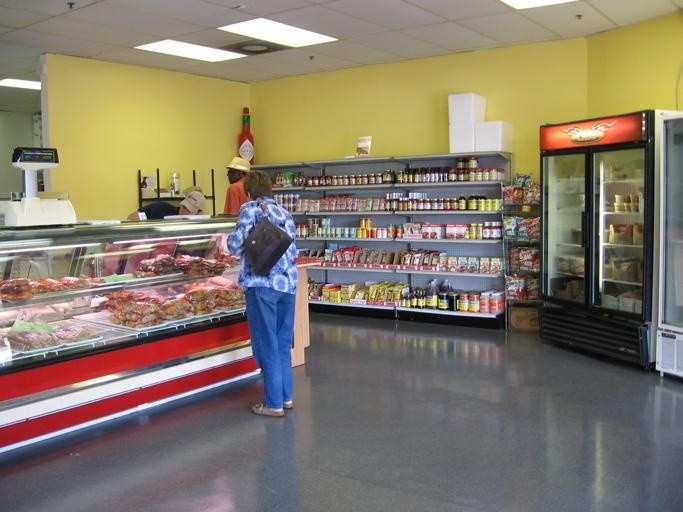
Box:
[221,157,251,215]
[226,170,294,418]
[127,190,209,222]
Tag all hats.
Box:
[226,157,250,173]
[180,189,207,215]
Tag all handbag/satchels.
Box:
[243,216,291,275]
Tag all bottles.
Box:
[481,221,501,241]
[405,289,425,309]
[238,106,253,166]
[458,193,476,211]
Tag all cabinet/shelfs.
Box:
[247,147,514,328]
[135,168,215,215]
[0,217,294,454]
[503,184,543,326]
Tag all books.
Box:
[356,134,372,158]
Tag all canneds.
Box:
[417,198,458,211]
[335,218,387,239]
[307,169,395,186]
[448,155,504,182]
[438,291,479,312]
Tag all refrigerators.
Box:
[655,112,683,381]
[539,108,654,371]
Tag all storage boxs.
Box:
[473,118,513,152]
[446,92,487,125]
[447,122,475,154]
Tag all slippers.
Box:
[252,401,294,417]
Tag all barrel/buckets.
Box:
[438,275,453,308]
[426,278,440,307]
